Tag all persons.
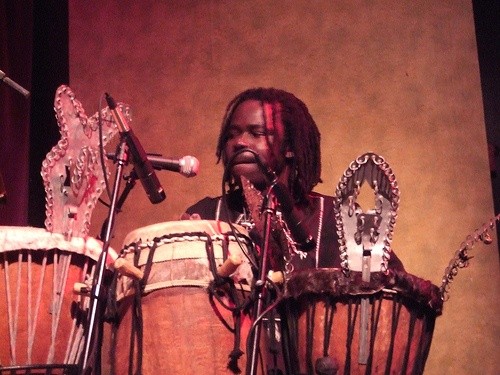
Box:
[179,87,406,375]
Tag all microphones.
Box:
[105,153,200,177]
[105,92,166,204]
[254,153,317,252]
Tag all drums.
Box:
[270,264,446,372]
[101,218,286,372]
[0,222,120,374]
